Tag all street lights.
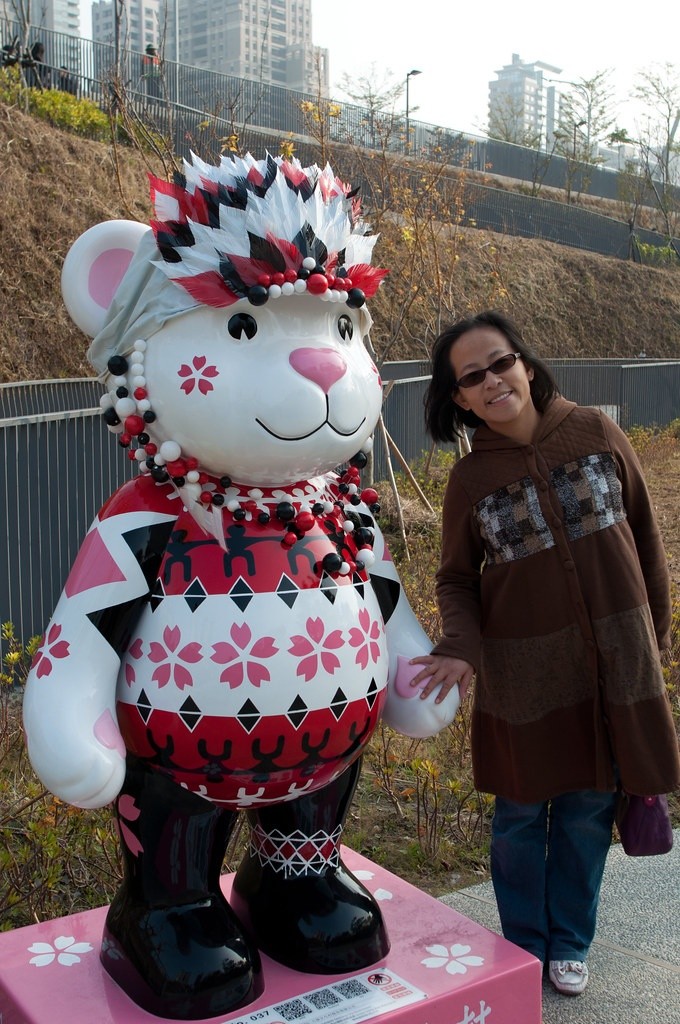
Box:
[405,70,422,155]
[572,120,588,159]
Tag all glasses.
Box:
[455,352,521,393]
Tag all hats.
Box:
[145,44,157,51]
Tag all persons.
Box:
[140,44,162,107]
[409,310,680,996]
[3,42,77,95]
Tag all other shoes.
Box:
[549,959,588,995]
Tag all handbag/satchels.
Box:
[615,794,673,856]
